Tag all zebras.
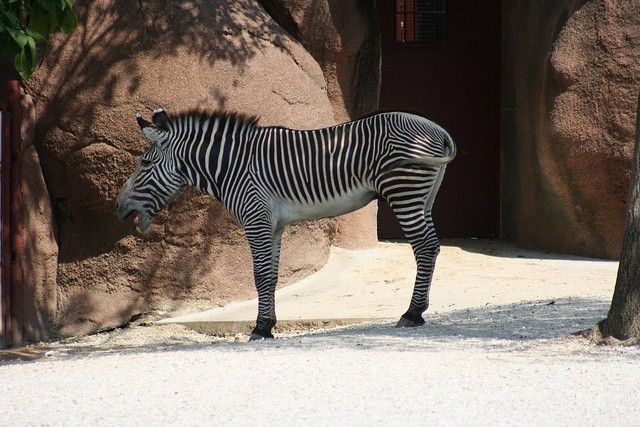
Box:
[115,106,457,341]
